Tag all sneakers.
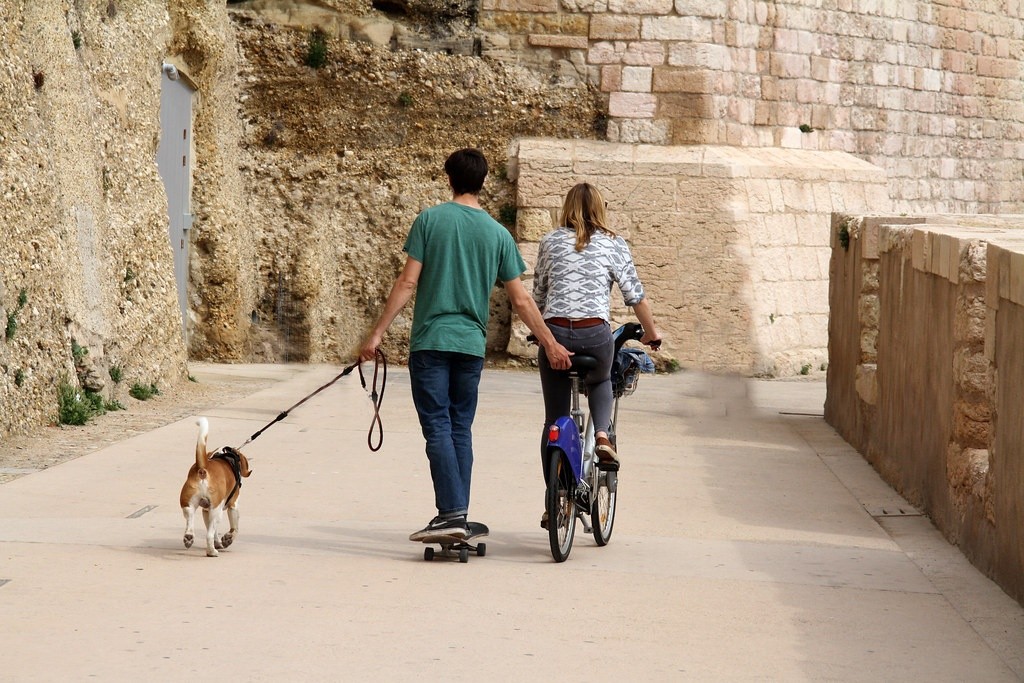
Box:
[542,511,554,521]
[408,515,472,541]
[595,437,620,468]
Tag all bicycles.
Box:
[525,322,662,563]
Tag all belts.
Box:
[548,317,603,328]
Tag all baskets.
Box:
[570,354,641,397]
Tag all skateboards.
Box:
[422,521,490,562]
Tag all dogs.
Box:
[179,416,252,557]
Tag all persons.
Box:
[532,182,661,531]
[360,144,575,541]
